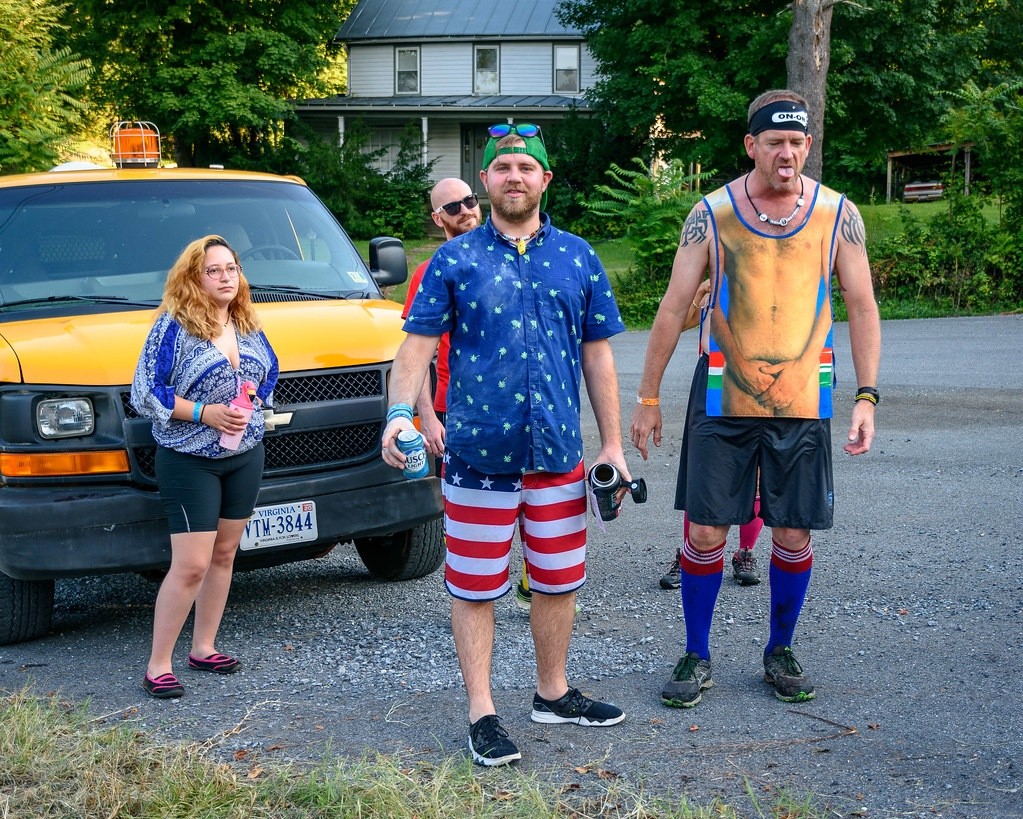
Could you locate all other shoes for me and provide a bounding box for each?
[188,653,242,674]
[515,580,580,615]
[144,672,185,697]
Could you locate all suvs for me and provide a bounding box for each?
[0,119,446,649]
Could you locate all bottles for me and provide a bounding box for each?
[588,462,647,521]
[219,381,257,451]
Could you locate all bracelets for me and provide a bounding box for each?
[192,401,206,424]
[636,395,660,406]
[386,403,414,423]
[854,387,880,405]
[691,296,707,309]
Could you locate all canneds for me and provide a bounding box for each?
[396,430,428,479]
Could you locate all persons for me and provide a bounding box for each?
[130,234,280,699]
[401,177,584,616]
[655,277,765,590]
[628,89,882,707]
[381,123,635,766]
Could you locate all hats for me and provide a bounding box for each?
[481,128,550,212]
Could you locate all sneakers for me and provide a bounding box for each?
[763,646,816,703]
[659,548,682,588]
[661,652,714,708]
[531,685,626,726]
[467,715,521,766]
[732,546,761,586]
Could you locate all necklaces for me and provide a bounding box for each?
[499,228,539,255]
[745,171,804,226]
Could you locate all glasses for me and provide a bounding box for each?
[434,193,478,216]
[203,263,242,280]
[484,123,545,148]
